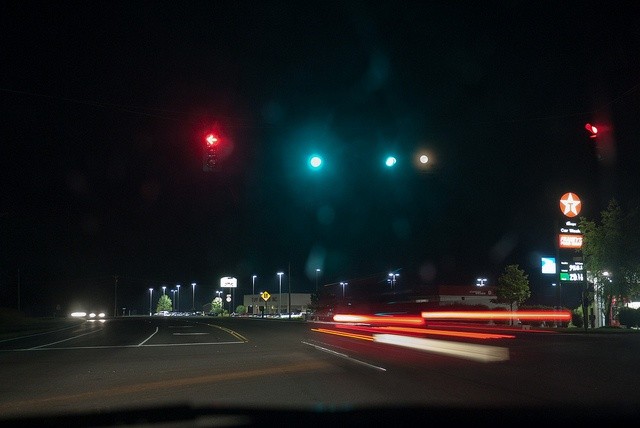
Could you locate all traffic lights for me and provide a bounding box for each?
[203,134,218,171]
[585,123,600,139]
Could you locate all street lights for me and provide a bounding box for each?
[277,272,284,304]
[253,276,257,302]
[149,288,153,317]
[192,283,196,308]
[340,282,348,298]
[316,269,321,291]
[171,290,177,309]
[162,287,166,295]
[176,285,180,311]
